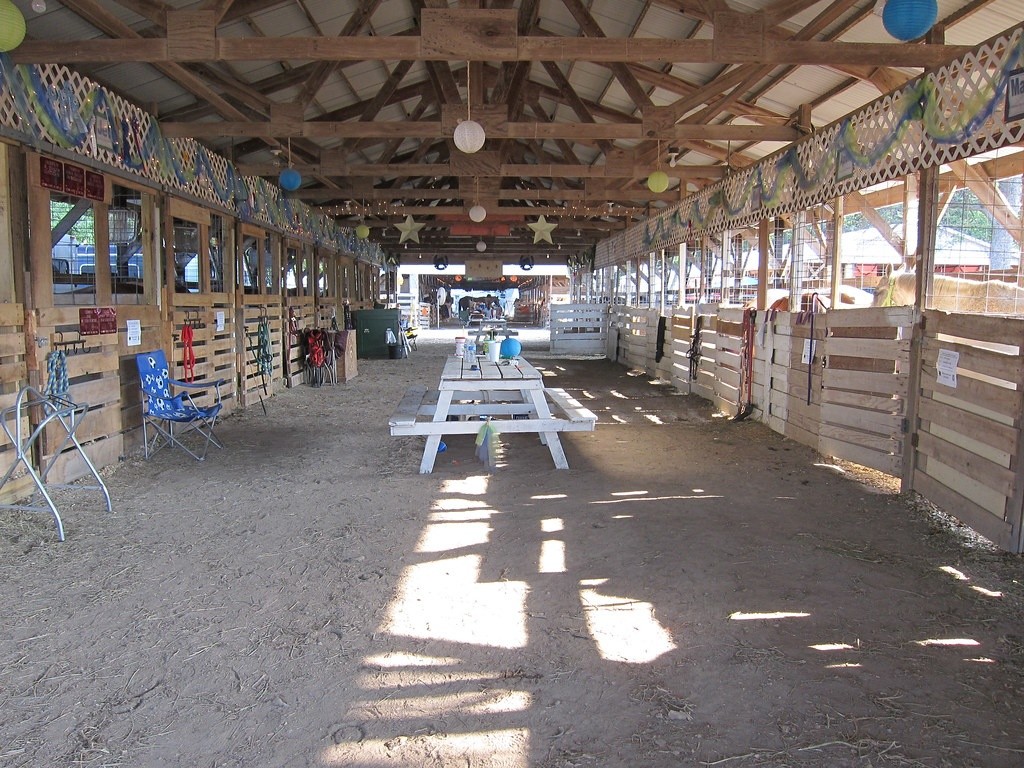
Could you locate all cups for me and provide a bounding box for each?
[455,337,466,356]
[488,341,501,362]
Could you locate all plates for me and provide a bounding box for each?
[454,353,503,359]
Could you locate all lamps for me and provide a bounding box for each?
[344,199,352,211]
[419,254,422,259]
[607,200,614,212]
[269,145,283,167]
[31,0,46,13]
[668,147,679,167]
[404,243,407,249]
[381,228,387,236]
[558,242,561,249]
[576,229,582,237]
[546,254,550,258]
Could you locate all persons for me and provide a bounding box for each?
[455,298,458,310]
[482,304,491,318]
[496,291,500,298]
[501,298,507,317]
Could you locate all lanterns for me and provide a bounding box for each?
[510,276,517,281]
[453,121,485,154]
[455,276,462,281]
[279,169,301,191]
[648,171,668,193]
[501,339,522,358]
[356,225,369,239]
[476,241,486,252]
[0,0,26,52]
[469,206,486,222]
[882,0,938,41]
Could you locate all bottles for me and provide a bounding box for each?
[463,338,476,363]
[482,334,490,353]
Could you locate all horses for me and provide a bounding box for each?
[869,261,1024,315]
[747,285,874,310]
[459,296,500,319]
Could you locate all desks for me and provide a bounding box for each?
[468,330,518,345]
[465,313,486,327]
[419,354,569,474]
[479,319,508,330]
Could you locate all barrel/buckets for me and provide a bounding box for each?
[388,345,402,359]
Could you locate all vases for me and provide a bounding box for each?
[387,345,404,359]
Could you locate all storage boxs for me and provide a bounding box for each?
[309,329,360,383]
[349,309,402,359]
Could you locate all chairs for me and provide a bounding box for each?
[136,350,225,461]
[408,334,417,351]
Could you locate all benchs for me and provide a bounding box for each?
[387,386,428,427]
[544,387,598,424]
